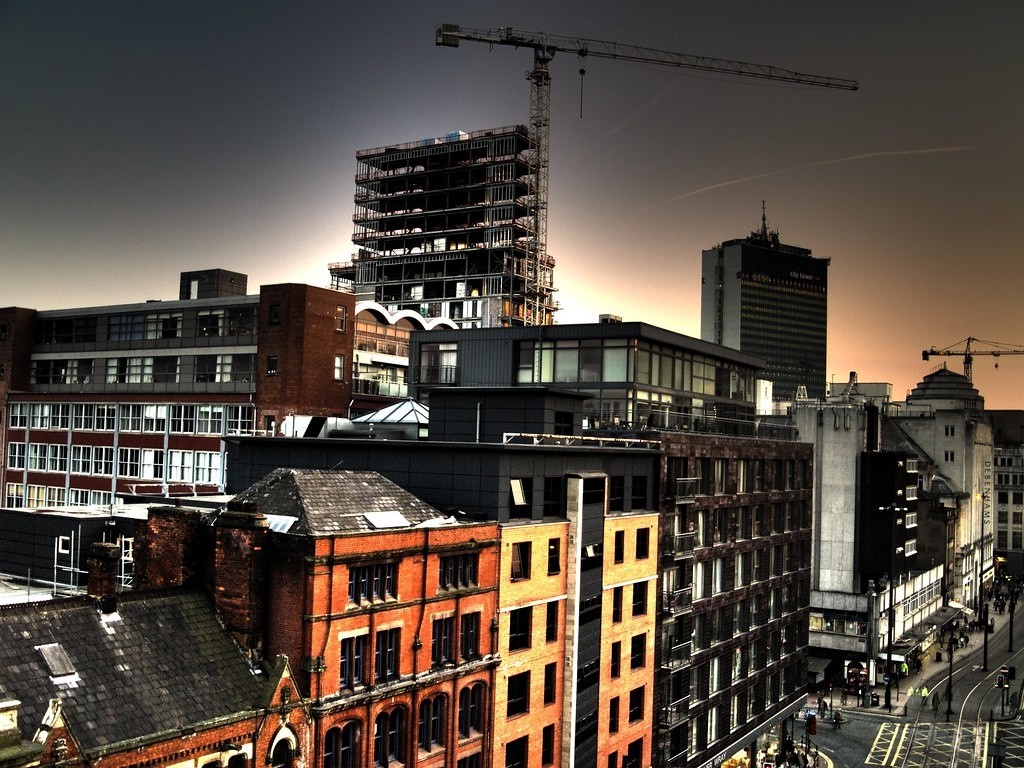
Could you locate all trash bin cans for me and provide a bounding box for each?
[935,651,943,662]
[871,693,880,706]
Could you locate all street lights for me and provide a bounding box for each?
[945,616,965,715]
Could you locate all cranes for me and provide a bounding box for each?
[432,23,863,325]
[922,334,1024,393]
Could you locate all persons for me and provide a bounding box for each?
[915,572,1024,674]
[762,686,848,768]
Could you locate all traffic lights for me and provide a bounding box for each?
[997,675,1004,689]
